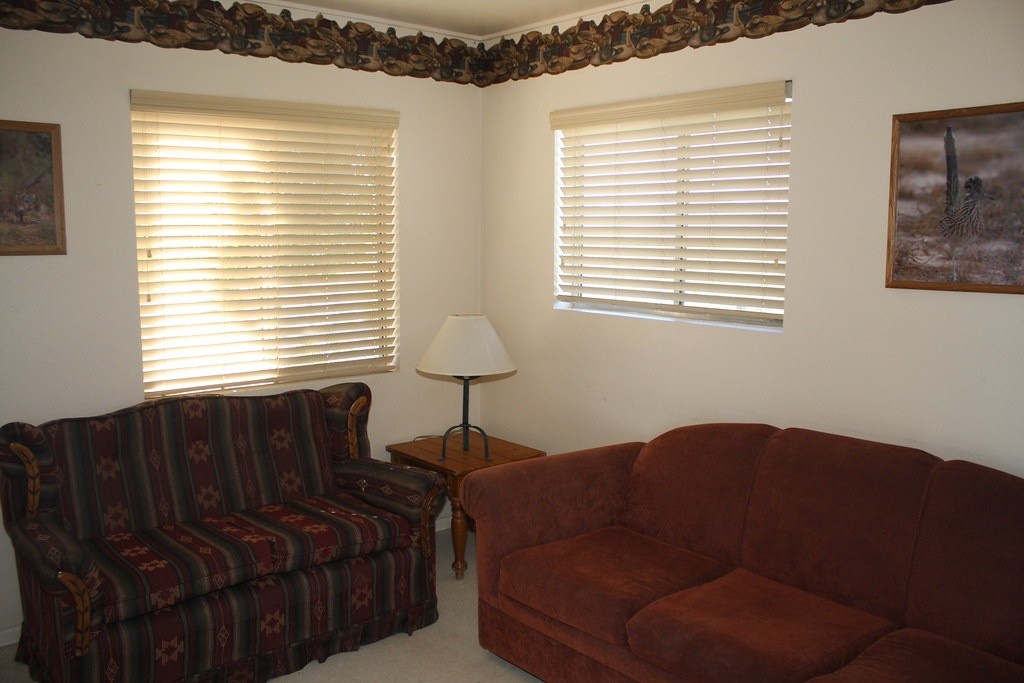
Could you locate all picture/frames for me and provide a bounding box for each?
[884,101,1024,296]
[0,119,68,257]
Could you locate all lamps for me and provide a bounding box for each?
[416,313,518,462]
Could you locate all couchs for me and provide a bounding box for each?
[456,423,1024,683]
[0,380,449,683]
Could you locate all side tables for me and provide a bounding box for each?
[387,430,548,580]
